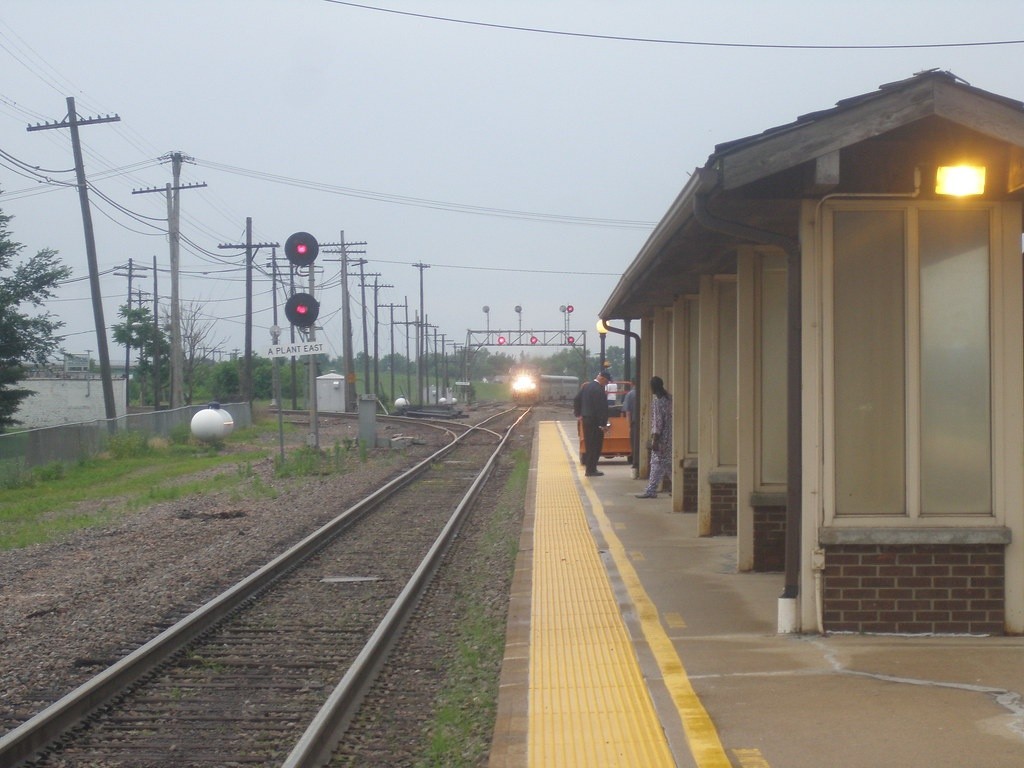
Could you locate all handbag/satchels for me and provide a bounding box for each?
[574,387,584,417]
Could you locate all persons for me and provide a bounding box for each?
[625,377,637,468]
[636,376,672,498]
[575,371,611,476]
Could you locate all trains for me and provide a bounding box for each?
[509,364,580,406]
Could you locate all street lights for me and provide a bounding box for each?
[596,319,610,392]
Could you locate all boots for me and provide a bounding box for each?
[631,467,639,480]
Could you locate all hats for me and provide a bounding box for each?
[599,371,611,381]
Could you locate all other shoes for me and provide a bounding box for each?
[585,470,604,477]
[634,492,657,498]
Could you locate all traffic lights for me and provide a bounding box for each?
[285,292,320,327]
[284,232,319,266]
